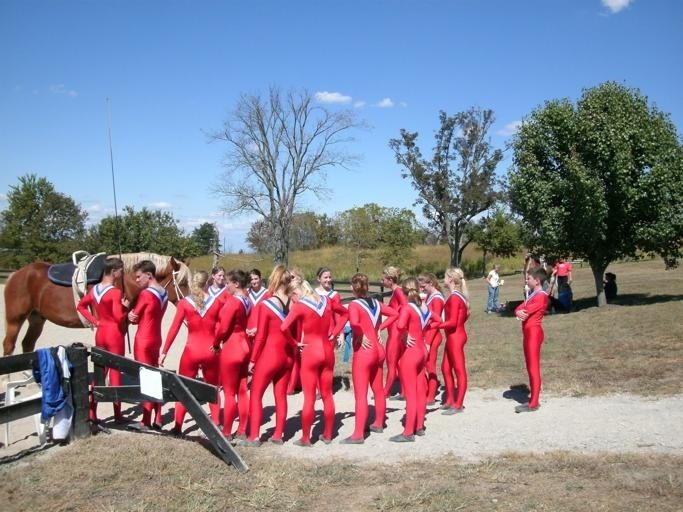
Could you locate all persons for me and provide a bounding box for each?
[127,261,169,431]
[158,265,349,443]
[79,260,132,426]
[515,268,548,413]
[419,272,445,411]
[595,273,617,302]
[389,278,442,442]
[524,252,573,314]
[340,274,399,443]
[381,266,408,398]
[486,264,502,314]
[439,267,469,415]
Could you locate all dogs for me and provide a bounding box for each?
[2,251,192,391]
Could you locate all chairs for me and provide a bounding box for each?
[366,423,382,432]
[115,415,163,434]
[389,396,404,401]
[317,432,331,444]
[264,436,283,445]
[515,401,541,413]
[440,402,463,415]
[293,439,311,445]
[167,428,182,438]
[219,424,261,447]
[390,428,424,442]
[340,437,365,443]
[91,418,101,434]
